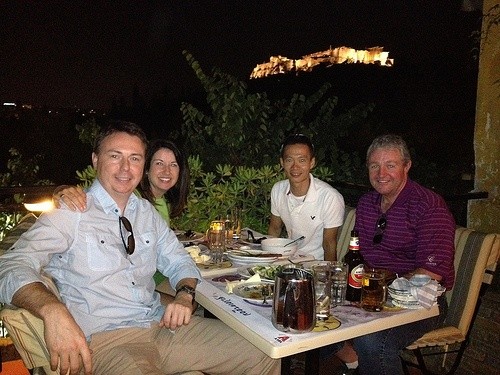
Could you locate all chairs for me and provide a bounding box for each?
[0,276,76,375]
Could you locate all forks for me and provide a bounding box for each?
[247,268,272,280]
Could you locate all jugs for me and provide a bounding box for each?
[271,268,316,334]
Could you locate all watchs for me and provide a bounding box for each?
[175,284,196,304]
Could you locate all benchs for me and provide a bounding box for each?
[336,205,500,375]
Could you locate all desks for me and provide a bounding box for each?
[173,228,440,358]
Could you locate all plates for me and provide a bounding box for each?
[227,236,278,265]
[174,231,205,243]
[301,260,331,271]
[232,282,274,306]
[236,263,278,282]
[182,243,208,254]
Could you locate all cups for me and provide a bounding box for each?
[206,221,225,252]
[360,268,387,311]
[222,207,241,243]
[327,261,348,306]
[313,266,331,320]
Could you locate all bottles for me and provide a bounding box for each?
[342,230,365,302]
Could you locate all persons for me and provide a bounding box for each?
[268,136,358,375]
[0,122,281,375]
[334,135,455,375]
[51,141,204,318]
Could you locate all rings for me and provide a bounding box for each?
[60,194,64,198]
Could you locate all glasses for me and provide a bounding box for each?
[373,217,387,245]
[119,216,135,255]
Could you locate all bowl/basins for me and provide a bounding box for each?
[260,237,298,259]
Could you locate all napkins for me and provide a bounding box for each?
[184,245,210,262]
[227,273,261,293]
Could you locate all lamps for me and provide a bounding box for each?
[23,194,52,212]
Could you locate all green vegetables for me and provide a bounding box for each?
[255,265,281,279]
